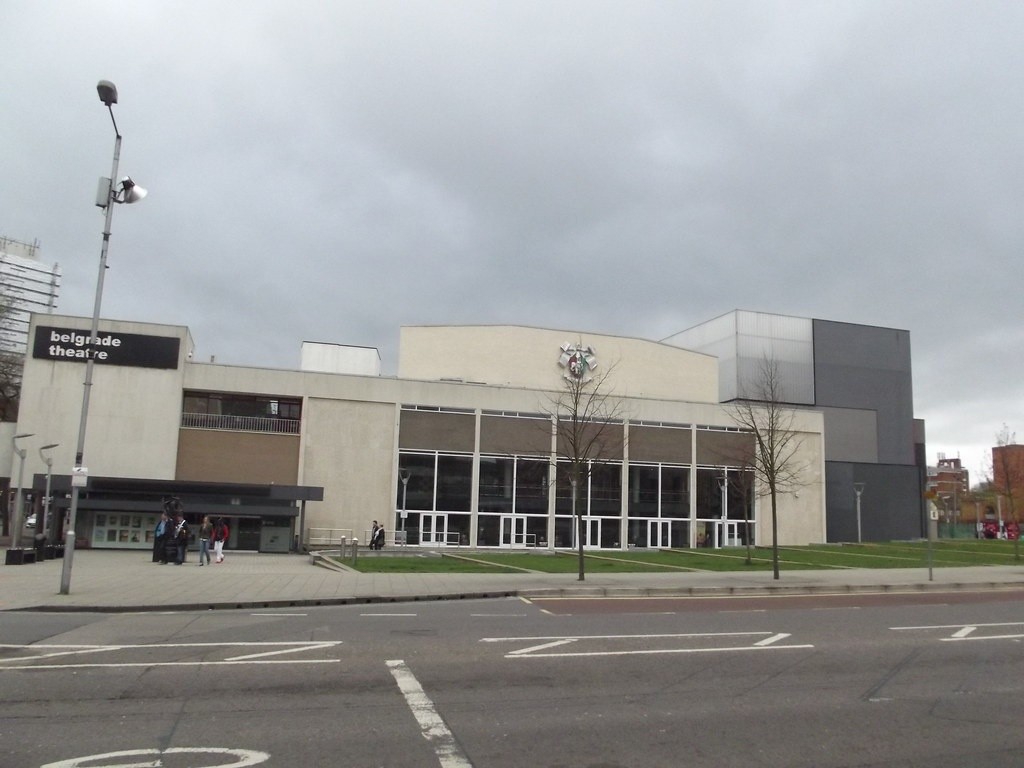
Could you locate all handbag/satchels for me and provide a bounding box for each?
[378,539,384,546]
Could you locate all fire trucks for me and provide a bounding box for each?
[976,520,1021,541]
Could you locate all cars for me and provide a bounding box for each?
[26,514,37,529]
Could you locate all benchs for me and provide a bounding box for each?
[45,545,65,560]
[5,548,37,565]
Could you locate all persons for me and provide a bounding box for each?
[153,512,189,565]
[198,516,213,565]
[212,516,228,563]
[369,520,385,550]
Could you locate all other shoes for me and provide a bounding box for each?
[160,561,168,565]
[215,561,221,563]
[199,564,204,566]
[221,556,224,561]
[175,561,182,565]
[207,560,210,565]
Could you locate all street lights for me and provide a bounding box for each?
[12,432,34,548]
[38,443,61,535]
[853,483,865,544]
[717,476,730,548]
[398,467,412,549]
[58,80,123,595]
